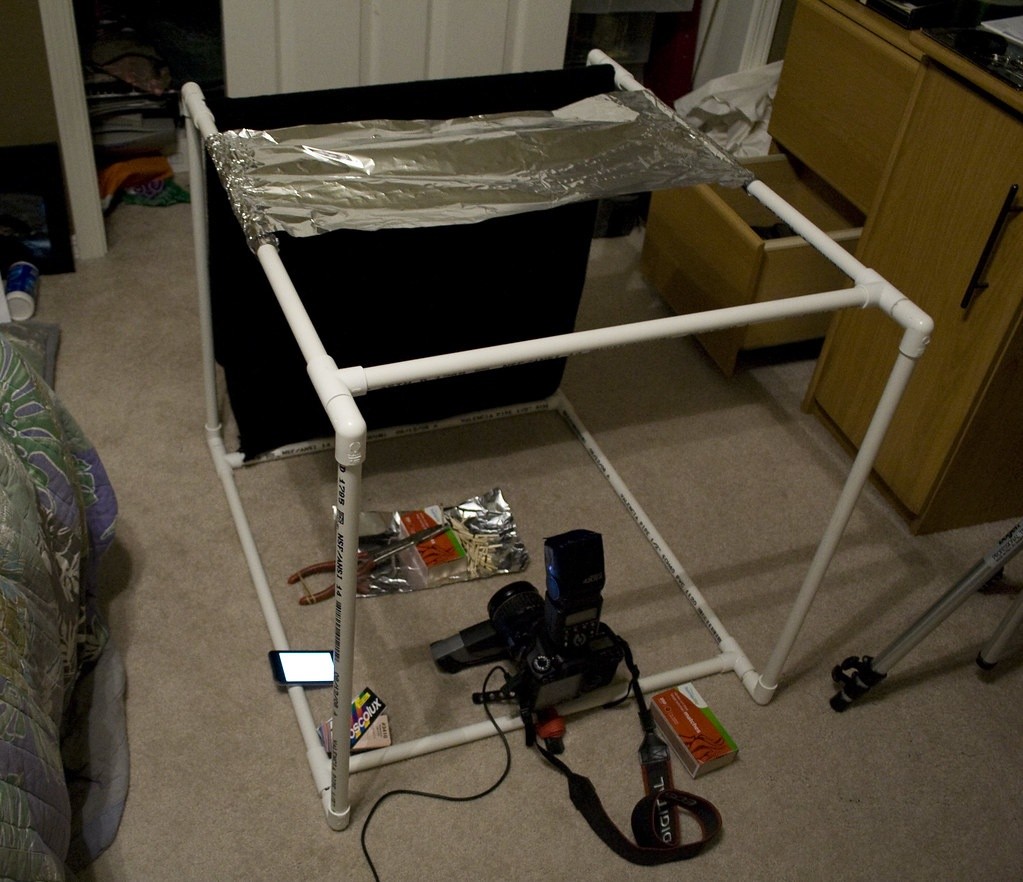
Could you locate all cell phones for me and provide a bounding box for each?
[268,650,335,687]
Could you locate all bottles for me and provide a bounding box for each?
[4,262,40,321]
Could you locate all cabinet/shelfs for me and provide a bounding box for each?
[639,0,1023,534]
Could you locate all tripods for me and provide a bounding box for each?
[829,525,1023,714]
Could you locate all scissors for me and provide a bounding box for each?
[287,521,450,606]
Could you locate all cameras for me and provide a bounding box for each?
[486,527,624,712]
